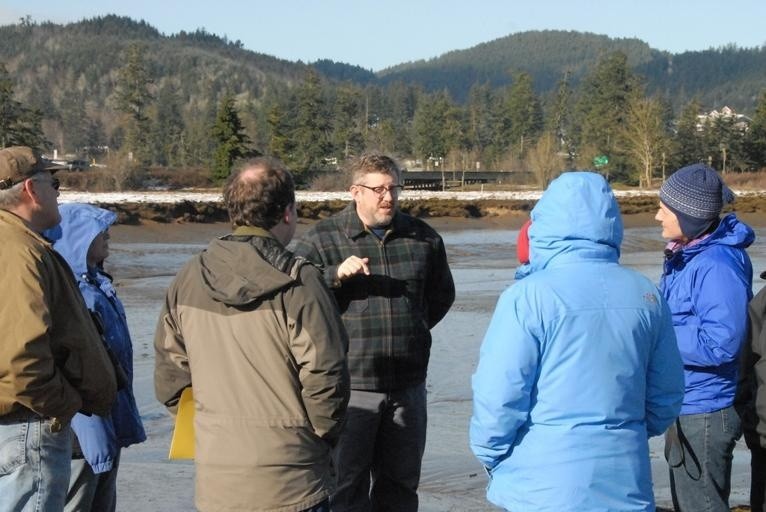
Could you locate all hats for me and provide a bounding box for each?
[660,164,734,240]
[0,146,70,191]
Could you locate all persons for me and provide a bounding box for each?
[155,162,350,512]
[291,154,455,511]
[48,203,147,512]
[0,146,127,512]
[469,170,685,511]
[733,270,766,510]
[653,161,757,512]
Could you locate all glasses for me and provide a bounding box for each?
[34,176,60,190]
[356,184,405,196]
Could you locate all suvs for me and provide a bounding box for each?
[62,158,90,174]
